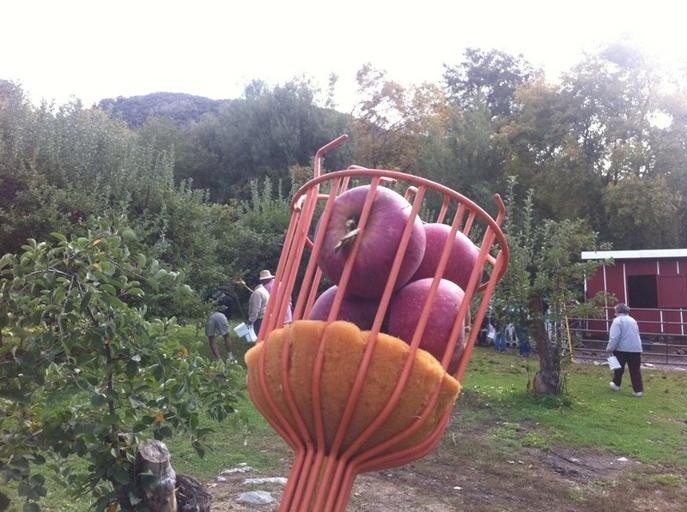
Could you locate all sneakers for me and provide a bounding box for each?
[632,392,642,396]
[609,381,620,391]
[494,347,531,358]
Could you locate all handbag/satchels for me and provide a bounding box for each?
[232,321,258,343]
[606,353,621,371]
[487,332,495,338]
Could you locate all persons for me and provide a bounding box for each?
[247,270,292,338]
[490,305,537,356]
[606,303,642,397]
[205,305,237,364]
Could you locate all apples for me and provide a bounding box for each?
[309,184,478,375]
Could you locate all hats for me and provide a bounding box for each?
[258,270,275,280]
